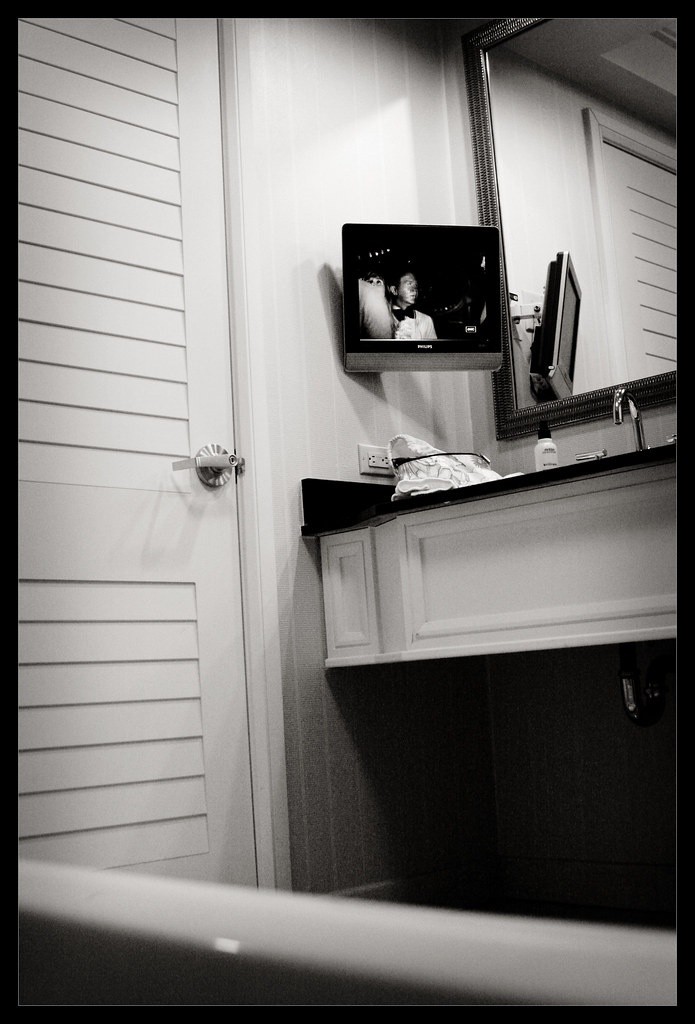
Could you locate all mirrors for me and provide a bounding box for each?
[463,17,677,443]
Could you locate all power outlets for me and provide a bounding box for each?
[358,443,399,477]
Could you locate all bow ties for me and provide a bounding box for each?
[391,304,414,322]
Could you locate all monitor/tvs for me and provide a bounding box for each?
[342,223,504,374]
[529,252,583,402]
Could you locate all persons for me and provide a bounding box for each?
[384,269,438,340]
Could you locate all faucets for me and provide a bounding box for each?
[612,387,646,453]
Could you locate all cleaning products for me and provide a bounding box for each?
[534,421,558,474]
